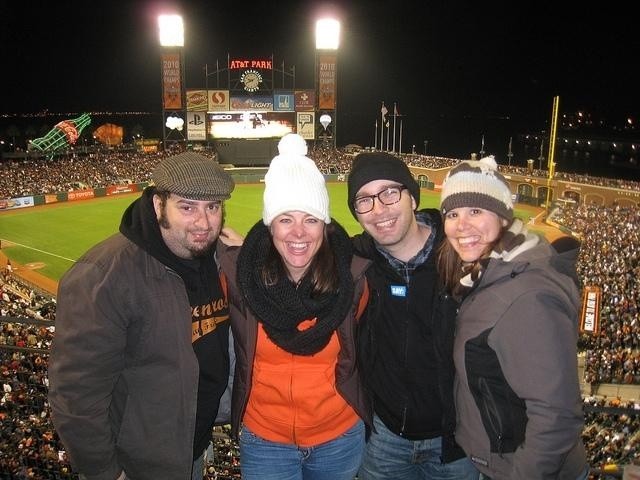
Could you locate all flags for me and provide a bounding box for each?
[380,104,388,117]
[395,107,400,117]
[385,122,389,127]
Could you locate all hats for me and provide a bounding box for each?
[261,132,331,227]
[347,151,422,222]
[150,150,235,202]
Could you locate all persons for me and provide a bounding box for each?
[550,197,640,479]
[217,153,480,479]
[253,113,261,128]
[0,136,216,194]
[48,153,236,480]
[438,156,589,478]
[306,143,353,174]
[394,152,460,168]
[242,109,250,128]
[497,165,639,191]
[214,133,377,478]
[0,260,79,480]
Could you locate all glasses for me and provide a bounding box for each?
[439,157,515,222]
[352,185,409,214]
